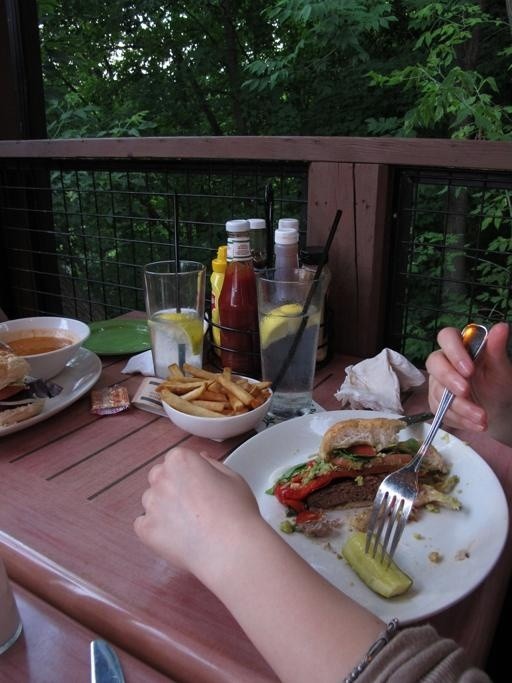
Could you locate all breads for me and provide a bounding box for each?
[321,417,406,458]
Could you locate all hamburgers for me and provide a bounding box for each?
[0,355,43,425]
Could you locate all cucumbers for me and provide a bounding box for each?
[342,533,411,598]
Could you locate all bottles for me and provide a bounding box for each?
[298,244,336,369]
[209,216,302,382]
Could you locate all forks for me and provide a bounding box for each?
[364,322,492,568]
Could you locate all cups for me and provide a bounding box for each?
[259,263,324,410]
[1,561,25,655]
[149,307,210,338]
[141,261,209,387]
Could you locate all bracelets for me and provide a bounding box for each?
[344,617,399,682]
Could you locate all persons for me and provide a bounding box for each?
[131,319,511,682]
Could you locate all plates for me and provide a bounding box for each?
[1,345,103,442]
[219,406,511,628]
[83,318,154,356]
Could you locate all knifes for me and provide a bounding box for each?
[87,638,124,683]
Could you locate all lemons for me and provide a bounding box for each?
[147,314,203,355]
[260,303,320,347]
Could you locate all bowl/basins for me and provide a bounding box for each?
[160,373,272,443]
[0,314,91,383]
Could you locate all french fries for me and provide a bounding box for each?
[154,361,272,417]
